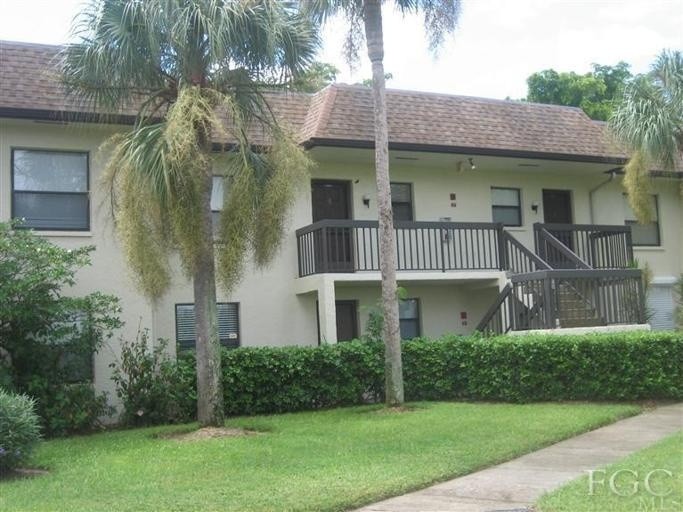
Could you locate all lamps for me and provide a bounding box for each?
[467,158,478,171]
[531,199,538,215]
[363,193,371,208]
